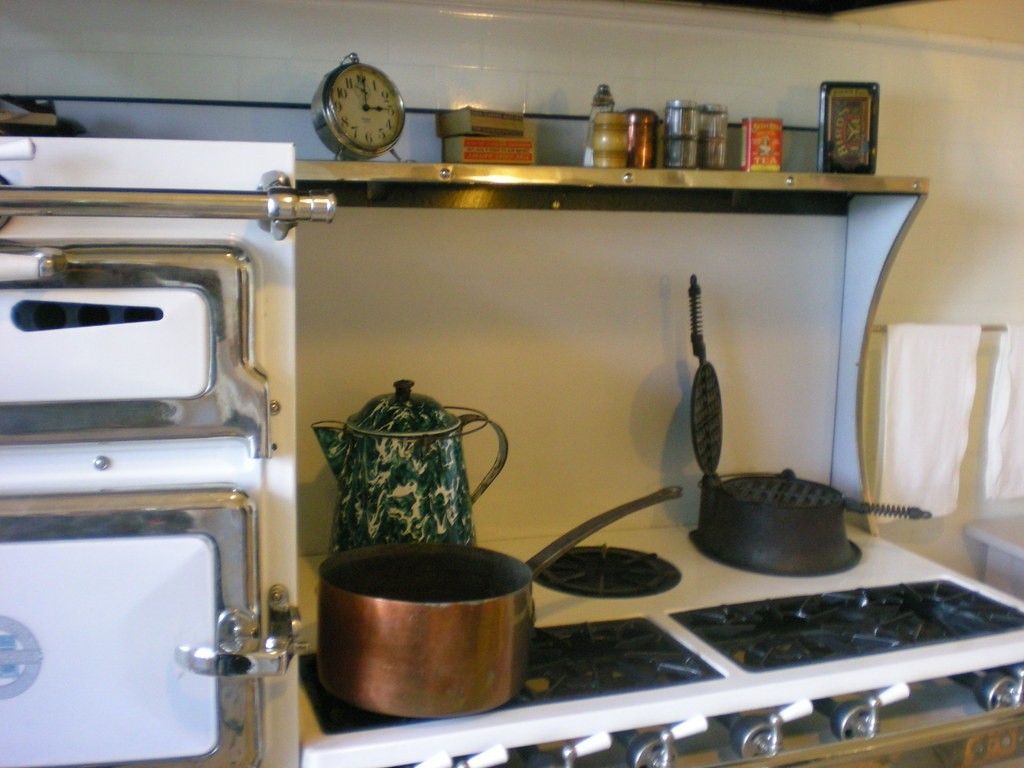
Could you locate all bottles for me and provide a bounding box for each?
[623,107,658,168]
[593,113,631,168]
[697,103,728,169]
[582,84,614,166]
[663,100,699,169]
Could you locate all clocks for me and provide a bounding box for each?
[310,53,406,161]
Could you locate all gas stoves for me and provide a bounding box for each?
[299,526,1024,768]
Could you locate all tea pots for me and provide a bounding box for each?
[310,379,508,546]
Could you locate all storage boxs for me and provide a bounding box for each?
[436,105,524,138]
[442,135,536,164]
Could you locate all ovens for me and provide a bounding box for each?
[1,135,337,768]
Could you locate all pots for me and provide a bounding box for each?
[315,483,681,719]
[686,273,932,575]
